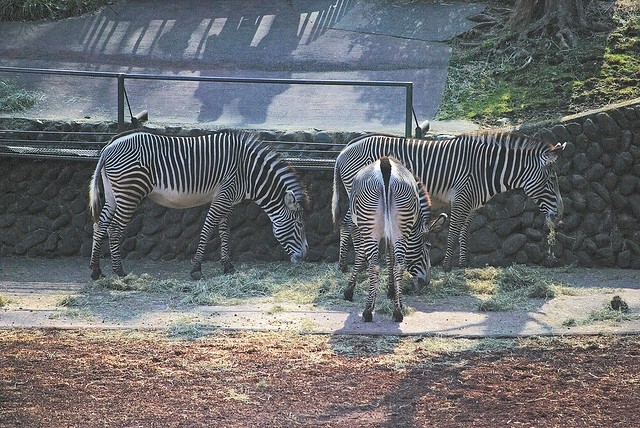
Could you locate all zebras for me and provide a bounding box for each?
[332,131,567,274]
[343,155,449,322]
[88,127,311,279]
